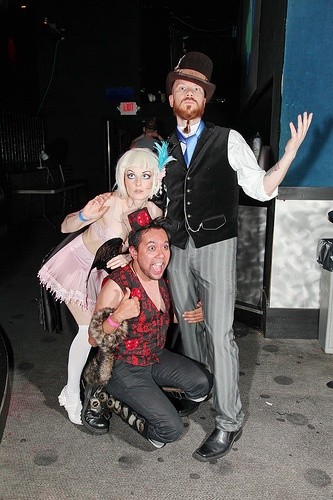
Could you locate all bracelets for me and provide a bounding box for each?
[78,210,88,222]
[107,316,121,328]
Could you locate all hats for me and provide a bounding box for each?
[165,52,216,104]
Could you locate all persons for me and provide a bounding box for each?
[37,131,211,449]
[149,52,313,461]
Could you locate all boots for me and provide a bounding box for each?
[162,385,207,402]
[80,385,166,449]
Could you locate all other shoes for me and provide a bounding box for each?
[57,385,84,426]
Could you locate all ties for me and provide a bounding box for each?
[174,120,205,169]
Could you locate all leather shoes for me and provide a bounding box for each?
[178,400,202,417]
[192,428,242,461]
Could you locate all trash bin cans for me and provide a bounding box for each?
[316,238,333,354]
